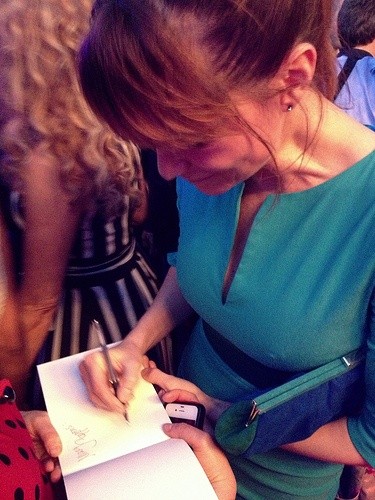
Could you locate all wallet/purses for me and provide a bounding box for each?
[218,346,366,458]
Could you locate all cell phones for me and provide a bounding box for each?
[163,400,204,452]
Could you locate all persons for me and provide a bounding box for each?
[0,0,236,500]
[329,0,375,131]
[74,0,375,500]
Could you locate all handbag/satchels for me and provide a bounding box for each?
[0,379,54,500]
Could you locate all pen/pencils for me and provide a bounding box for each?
[90,319,131,422]
